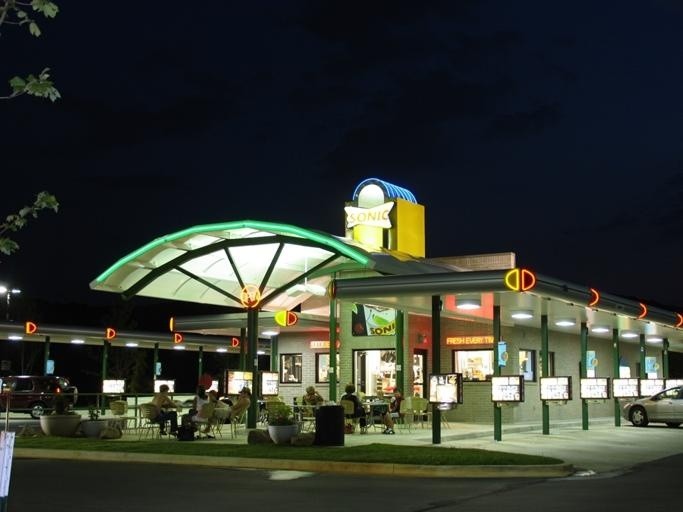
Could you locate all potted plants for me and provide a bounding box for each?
[260,403,300,445]
[39,391,82,437]
[78,405,108,437]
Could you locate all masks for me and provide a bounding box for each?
[394,393,400,398]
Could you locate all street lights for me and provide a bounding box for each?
[0,286,21,322]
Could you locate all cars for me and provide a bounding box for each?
[622,384,683,430]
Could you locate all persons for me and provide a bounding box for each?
[341,384,367,434]
[149,385,179,436]
[303,386,323,417]
[183,385,251,433]
[383,388,406,434]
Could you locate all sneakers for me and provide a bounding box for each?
[385,427,392,433]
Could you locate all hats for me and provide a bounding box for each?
[206,390,217,397]
[239,387,250,393]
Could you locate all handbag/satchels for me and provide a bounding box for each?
[176,426,197,441]
[344,423,355,434]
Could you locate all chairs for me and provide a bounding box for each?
[109,400,135,435]
[115,400,137,433]
[291,389,449,436]
[136,396,249,448]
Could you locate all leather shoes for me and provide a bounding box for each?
[171,431,177,436]
[160,431,167,435]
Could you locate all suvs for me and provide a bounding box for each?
[1,375,78,420]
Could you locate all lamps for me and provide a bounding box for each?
[454,297,481,311]
[509,309,535,321]
[620,329,638,338]
[645,334,664,343]
[591,324,610,334]
[552,316,576,327]
[285,247,327,298]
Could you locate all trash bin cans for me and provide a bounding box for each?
[314,406,344,445]
